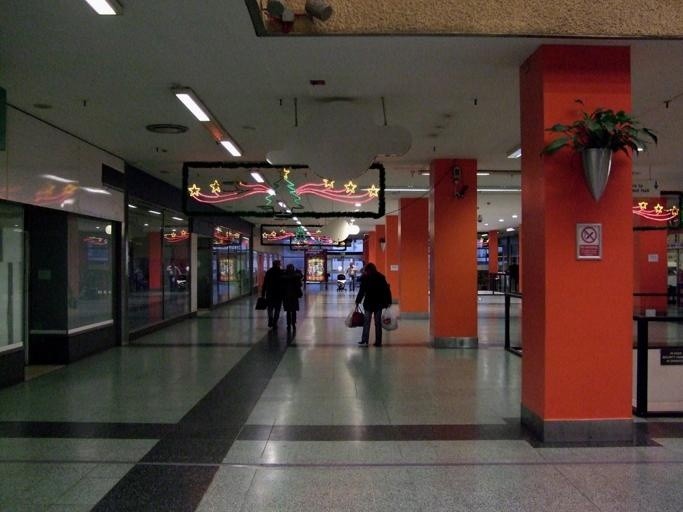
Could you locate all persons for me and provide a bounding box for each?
[281,264,303,329]
[262,260,284,329]
[355,263,392,347]
[507,258,519,293]
[346,263,357,292]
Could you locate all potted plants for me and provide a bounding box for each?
[540,107,659,201]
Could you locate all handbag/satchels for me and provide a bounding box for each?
[297,288,303,298]
[352,312,364,327]
[256,297,266,309]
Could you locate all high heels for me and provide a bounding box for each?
[358,339,381,347]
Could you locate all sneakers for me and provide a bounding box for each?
[268,318,277,327]
[288,321,295,329]
